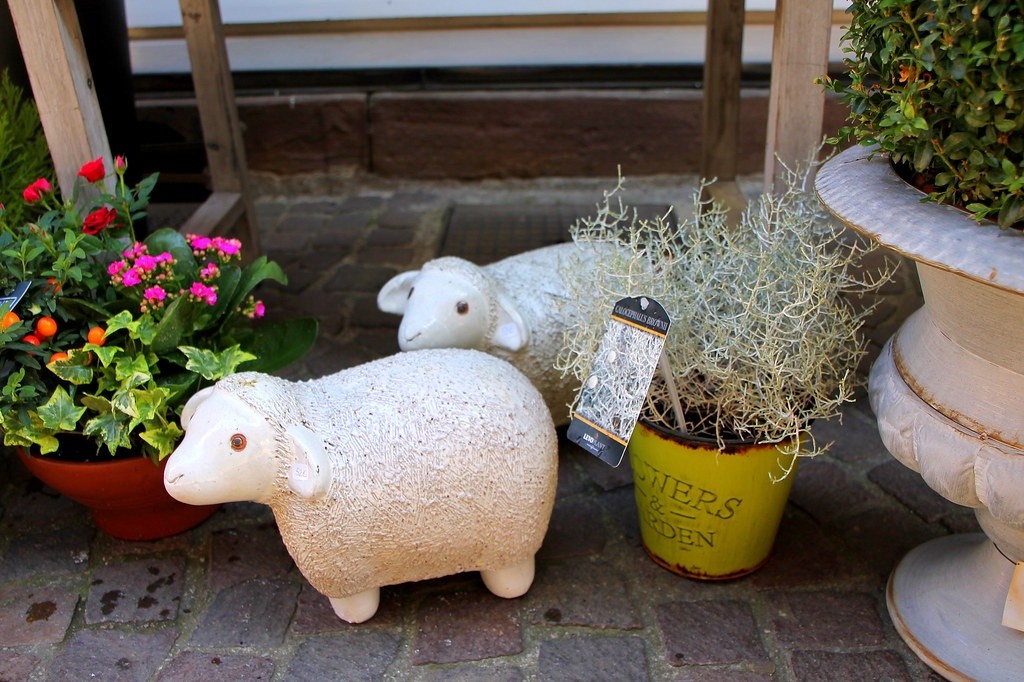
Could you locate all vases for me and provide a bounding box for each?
[14,438,225,545]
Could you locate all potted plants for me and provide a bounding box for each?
[537,131,901,585]
[815,0,1024,682]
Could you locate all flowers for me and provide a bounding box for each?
[0,153,318,470]
